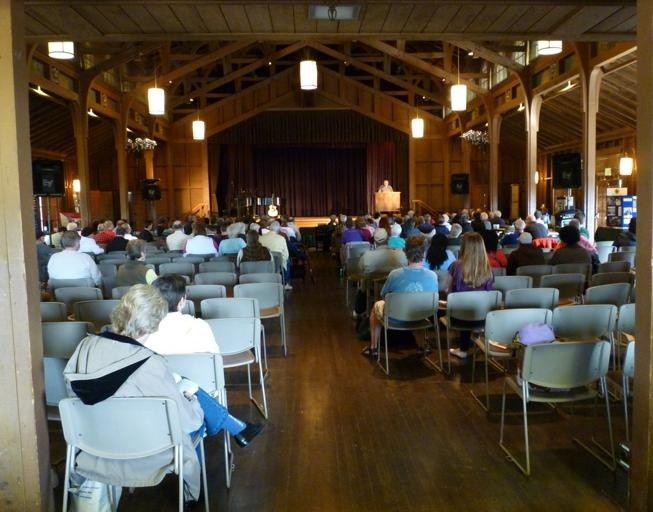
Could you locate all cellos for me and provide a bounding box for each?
[268,193,279,218]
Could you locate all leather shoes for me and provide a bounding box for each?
[234,420,264,447]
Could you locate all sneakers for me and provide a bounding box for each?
[284,283,292,291]
[449,347,467,359]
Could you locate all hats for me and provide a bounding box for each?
[372,228,387,243]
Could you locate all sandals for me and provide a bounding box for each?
[360,345,377,359]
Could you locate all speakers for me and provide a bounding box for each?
[33,160,64,196]
[143,179,160,200]
[452,173,469,194]
[553,153,581,188]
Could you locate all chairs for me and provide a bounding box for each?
[498,342,617,474]
[203,318,269,421]
[39,319,94,423]
[56,395,209,512]
[316,212,634,411]
[592,339,635,470]
[156,352,234,490]
[37,213,289,417]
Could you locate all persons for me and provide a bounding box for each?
[594,216,636,248]
[327,207,551,355]
[60,282,265,512]
[137,273,220,354]
[155,213,302,290]
[549,212,599,276]
[36,219,158,291]
[376,179,394,192]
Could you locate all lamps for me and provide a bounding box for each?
[190,101,205,140]
[449,47,468,112]
[71,179,80,192]
[299,47,318,92]
[146,65,166,116]
[44,40,76,61]
[536,41,562,56]
[410,98,424,139]
[619,156,633,176]
